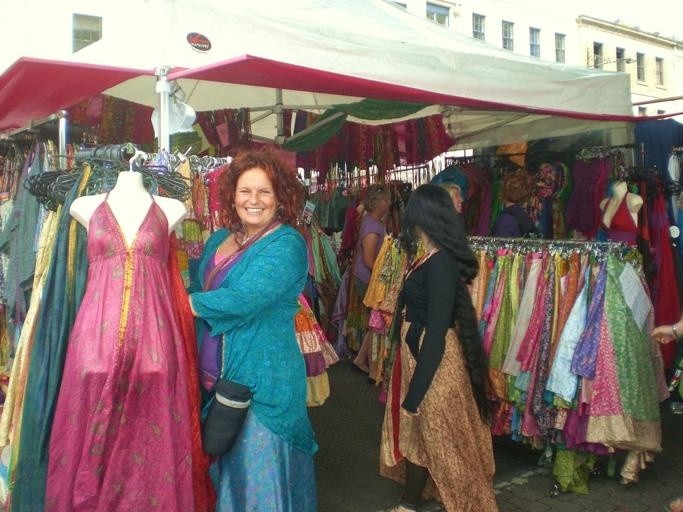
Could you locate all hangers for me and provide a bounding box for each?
[21,141,227,213]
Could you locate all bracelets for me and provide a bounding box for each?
[672,325,681,340]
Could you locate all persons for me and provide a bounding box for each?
[351,184,391,379]
[600,182,643,244]
[387,184,498,512]
[183,154,319,512]
[45,171,215,512]
[651,318,683,512]
